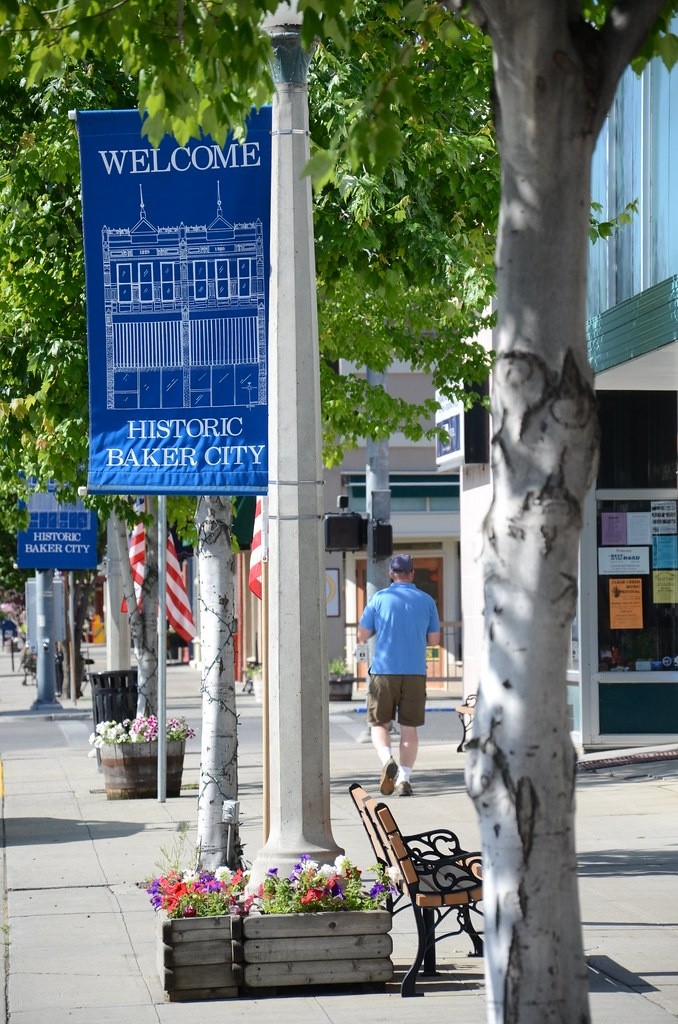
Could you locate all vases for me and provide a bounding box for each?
[100,740,186,800]
[243,904,393,988]
[158,911,244,1003]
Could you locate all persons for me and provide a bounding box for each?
[359,553,441,796]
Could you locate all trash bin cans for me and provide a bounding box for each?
[55,651,64,695]
[86,670,139,773]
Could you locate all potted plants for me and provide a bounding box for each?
[326,658,353,702]
[244,665,264,702]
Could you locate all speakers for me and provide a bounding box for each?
[324,514,364,551]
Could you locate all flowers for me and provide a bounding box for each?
[242,853,398,914]
[135,833,248,919]
[87,715,196,748]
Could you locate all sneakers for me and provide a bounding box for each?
[380,755,398,796]
[395,781,411,796]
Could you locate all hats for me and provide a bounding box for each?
[390,553,412,570]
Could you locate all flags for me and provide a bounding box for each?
[248,495,263,602]
[120,496,196,641]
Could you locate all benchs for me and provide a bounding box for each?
[349,782,487,998]
